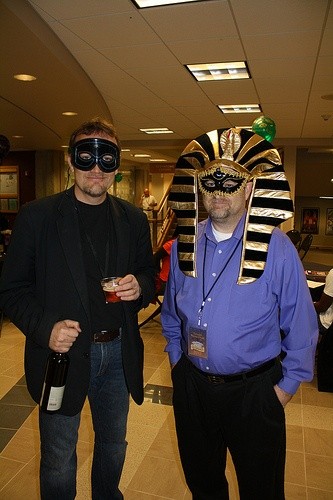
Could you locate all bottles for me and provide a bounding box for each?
[40,352,68,413]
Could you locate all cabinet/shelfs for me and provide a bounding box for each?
[0,165,35,225]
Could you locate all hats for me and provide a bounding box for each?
[167,128,295,285]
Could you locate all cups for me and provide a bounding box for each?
[101,277,121,303]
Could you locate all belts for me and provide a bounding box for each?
[185,353,281,385]
[91,327,121,343]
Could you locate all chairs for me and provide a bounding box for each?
[286,230,313,261]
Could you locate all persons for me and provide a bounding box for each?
[154,237,177,296]
[314,266,333,330]
[159,127,319,500]
[0,116,158,500]
[138,184,157,241]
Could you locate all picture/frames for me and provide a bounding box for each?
[325,208,333,236]
[301,207,320,234]
